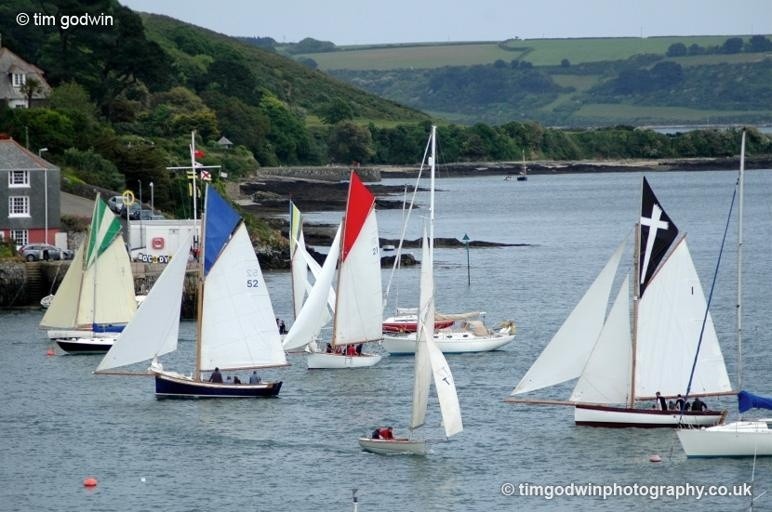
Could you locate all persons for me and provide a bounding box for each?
[372,426,393,440]
[326,342,362,355]
[250,371,260,384]
[651,392,707,411]
[208,367,241,384]
[275,318,285,334]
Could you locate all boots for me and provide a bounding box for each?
[18,241,73,261]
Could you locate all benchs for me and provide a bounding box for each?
[187,150,227,198]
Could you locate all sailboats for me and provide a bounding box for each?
[42,121,771,459]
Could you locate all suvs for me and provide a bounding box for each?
[18,241,73,261]
